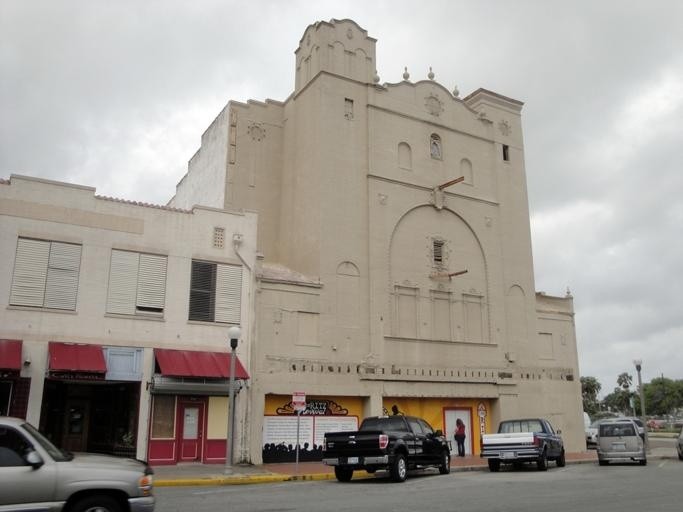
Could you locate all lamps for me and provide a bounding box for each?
[24,355,31,364]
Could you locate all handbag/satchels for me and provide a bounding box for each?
[454,434,466,442]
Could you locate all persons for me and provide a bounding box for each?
[453,418,465,456]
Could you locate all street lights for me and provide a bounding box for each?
[632,358,649,454]
[223,326,241,475]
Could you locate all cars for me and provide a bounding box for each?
[585,417,646,465]
[0,417,154,511]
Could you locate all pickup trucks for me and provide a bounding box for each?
[479,418,565,470]
[322,414,451,482]
